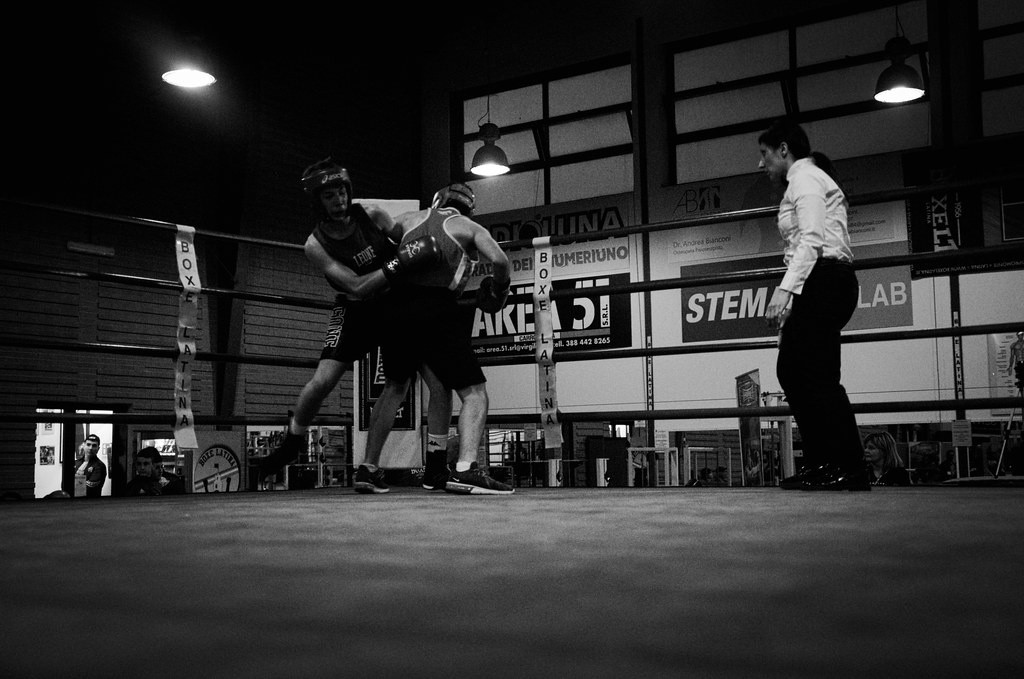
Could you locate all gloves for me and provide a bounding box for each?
[479,275,511,313]
[383,236,442,282]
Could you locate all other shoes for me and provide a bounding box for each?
[779,463,873,492]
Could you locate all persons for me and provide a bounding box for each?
[759,121,875,492]
[45,435,185,498]
[606,441,729,487]
[258,158,456,490]
[46,448,55,466]
[863,429,913,487]
[355,184,515,494]
[746,450,780,487]
[937,450,976,487]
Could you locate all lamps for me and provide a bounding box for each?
[469,67,511,178]
[161,32,218,88]
[873,0,927,104]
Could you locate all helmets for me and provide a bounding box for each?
[299,159,352,197]
[430,183,477,213]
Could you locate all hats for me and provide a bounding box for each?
[85,434,101,445]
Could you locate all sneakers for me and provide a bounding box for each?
[422,469,474,492]
[266,437,308,471]
[447,462,517,495]
[354,464,390,494]
